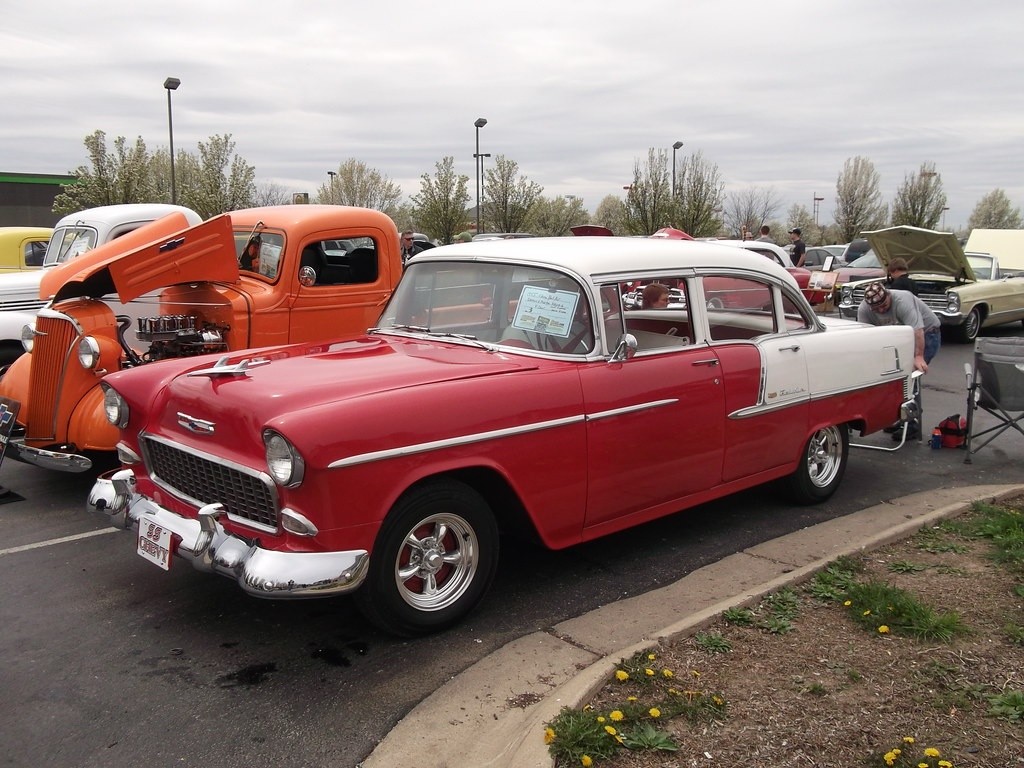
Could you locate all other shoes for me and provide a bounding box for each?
[891,424,919,439]
[884,420,901,433]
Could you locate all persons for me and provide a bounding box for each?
[641,283,670,309]
[754,226,777,259]
[884,258,919,295]
[857,282,942,442]
[399,230,424,270]
[451,232,481,305]
[788,227,806,267]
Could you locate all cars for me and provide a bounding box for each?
[836,224,1024,344]
[86,231,923,643]
[0,203,521,472]
[0,226,82,275]
[0,203,204,374]
[255,217,888,313]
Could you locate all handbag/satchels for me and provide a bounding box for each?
[939,414,967,448]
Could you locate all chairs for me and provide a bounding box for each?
[962,335,1024,463]
[348,247,378,284]
[626,329,688,351]
[302,245,327,285]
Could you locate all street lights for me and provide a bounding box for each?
[474,153,491,234]
[941,206,949,232]
[474,117,487,235]
[327,171,337,205]
[671,141,684,199]
[163,76,182,204]
[813,197,824,226]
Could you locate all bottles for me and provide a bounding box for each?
[933,427,941,436]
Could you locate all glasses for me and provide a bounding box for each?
[404,237,414,241]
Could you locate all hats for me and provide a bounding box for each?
[788,228,801,235]
[865,282,886,306]
[454,232,472,242]
